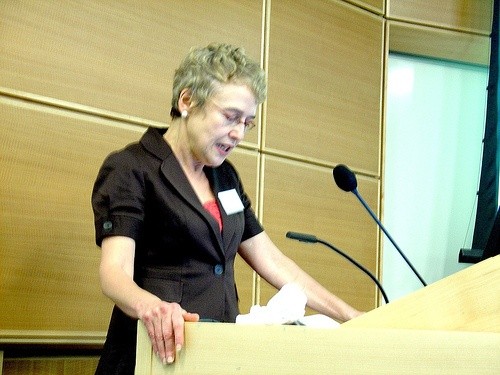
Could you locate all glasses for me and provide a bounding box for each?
[206,99,256,131]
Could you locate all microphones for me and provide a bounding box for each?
[333,164,428,287]
[286,231,390,304]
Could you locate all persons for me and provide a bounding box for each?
[91,43,369,375]
